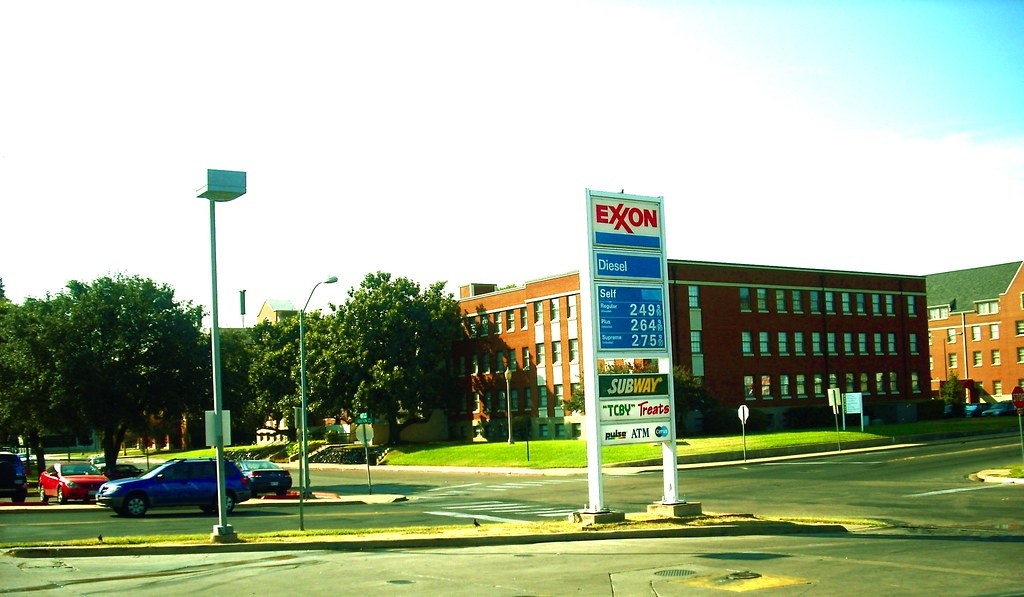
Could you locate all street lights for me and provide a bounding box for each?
[504,366,514,443]
[300,275,338,500]
[944,332,964,382]
[195,167,246,544]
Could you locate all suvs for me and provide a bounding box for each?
[94,457,252,518]
[0,452,28,503]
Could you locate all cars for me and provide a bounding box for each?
[233,460,292,498]
[982,401,1017,417]
[39,461,112,506]
[90,458,145,482]
[17,453,35,465]
[964,403,991,418]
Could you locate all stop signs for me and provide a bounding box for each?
[1012,386,1024,408]
